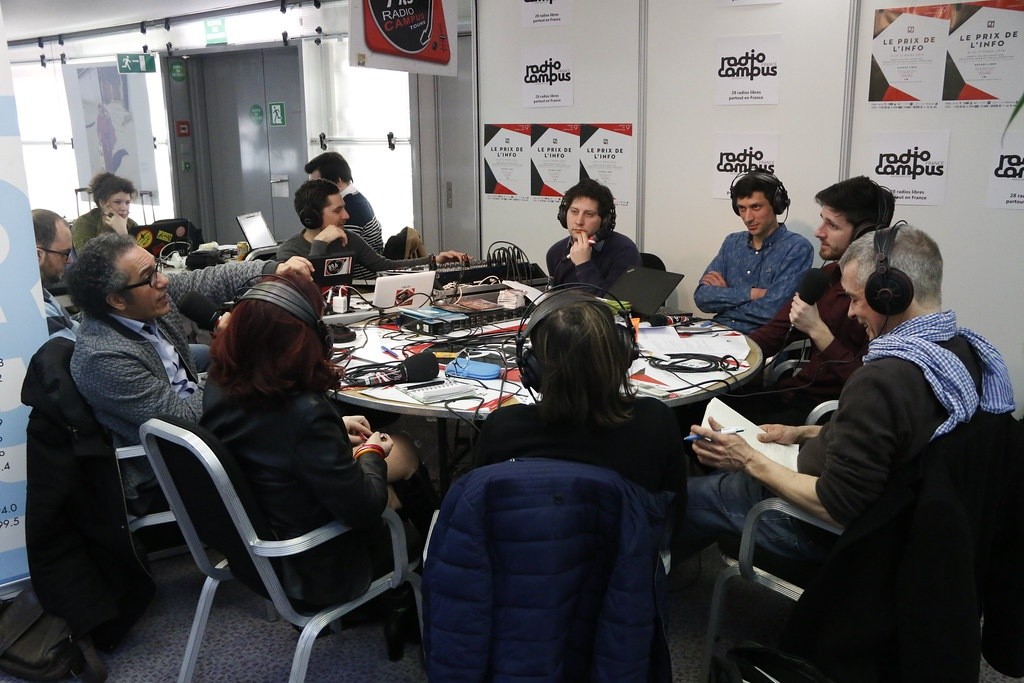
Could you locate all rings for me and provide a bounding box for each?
[108,213,113,217]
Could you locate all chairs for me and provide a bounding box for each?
[138,412,424,683]
[767,359,839,426]
[19,334,276,656]
[420,456,690,683]
[698,411,1024,683]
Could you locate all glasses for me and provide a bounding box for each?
[444,350,507,389]
[121,261,162,291]
[37,247,71,261]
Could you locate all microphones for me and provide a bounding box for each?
[649,314,704,327]
[562,229,613,263]
[177,292,226,340]
[784,268,829,343]
[349,352,440,387]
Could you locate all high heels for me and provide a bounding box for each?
[384,590,421,662]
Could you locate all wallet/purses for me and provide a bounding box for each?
[445,357,500,380]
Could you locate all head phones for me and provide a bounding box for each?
[514,283,640,394]
[209,274,336,363]
[730,169,790,216]
[848,180,894,241]
[299,179,322,229]
[557,186,616,231]
[865,220,914,316]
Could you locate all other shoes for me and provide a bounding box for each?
[717,533,766,560]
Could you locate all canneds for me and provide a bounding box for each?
[236,241,249,261]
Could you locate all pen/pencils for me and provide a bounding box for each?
[380,345,399,359]
[403,380,445,391]
[683,427,745,440]
[701,319,717,327]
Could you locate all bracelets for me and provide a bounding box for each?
[354,443,385,460]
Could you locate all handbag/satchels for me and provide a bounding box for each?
[0,589,107,683]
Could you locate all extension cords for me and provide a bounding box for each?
[320,309,380,325]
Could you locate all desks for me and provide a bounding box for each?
[327,316,765,503]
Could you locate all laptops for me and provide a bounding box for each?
[600,264,694,321]
[303,250,353,314]
[236,211,278,251]
[354,270,436,312]
[129,220,190,262]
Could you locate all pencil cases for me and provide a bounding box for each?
[445,358,501,379]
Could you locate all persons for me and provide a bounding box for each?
[31,209,80,341]
[475,284,688,548]
[276,179,473,295]
[656,167,1015,570]
[71,174,136,259]
[69,232,317,556]
[199,272,438,662]
[545,179,642,297]
[304,152,384,280]
